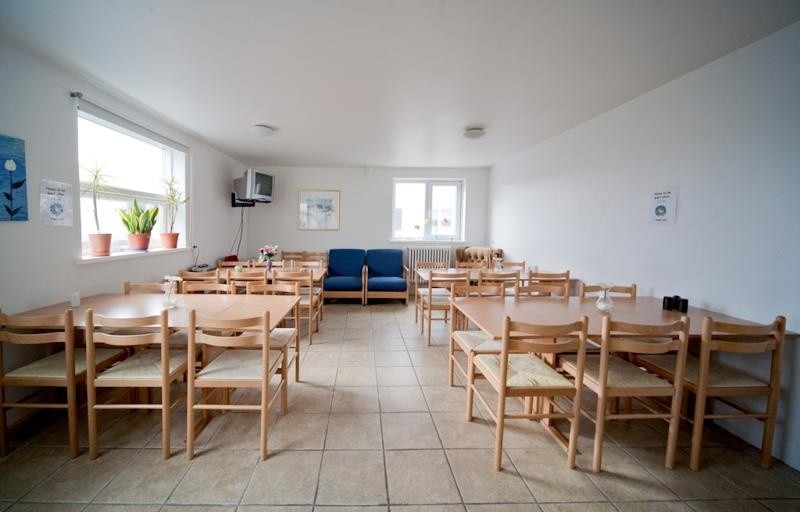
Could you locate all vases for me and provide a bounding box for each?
[265,257,272,271]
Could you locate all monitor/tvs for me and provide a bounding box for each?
[232,168,276,203]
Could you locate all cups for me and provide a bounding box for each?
[70,290,80,307]
[282,260,293,271]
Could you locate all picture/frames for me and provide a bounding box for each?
[298,188,340,230]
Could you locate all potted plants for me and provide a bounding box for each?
[87,169,112,257]
[158,174,190,248]
[118,198,159,250]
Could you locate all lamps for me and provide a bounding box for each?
[250,123,274,139]
[462,128,486,140]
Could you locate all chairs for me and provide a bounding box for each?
[558,316,691,472]
[635,315,787,471]
[528,269,570,297]
[122,280,178,295]
[0,309,126,460]
[473,316,590,473]
[414,262,448,323]
[178,269,221,295]
[86,308,200,460]
[290,259,324,269]
[241,281,300,384]
[580,282,636,418]
[281,250,305,262]
[477,270,520,298]
[252,259,285,269]
[216,259,251,268]
[454,260,491,269]
[186,307,288,461]
[513,282,570,415]
[365,249,410,306]
[323,248,365,305]
[181,280,236,294]
[448,283,506,422]
[226,269,267,296]
[304,252,328,263]
[498,261,526,270]
[418,270,470,346]
[271,269,320,346]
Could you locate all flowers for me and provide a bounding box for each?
[258,245,280,259]
